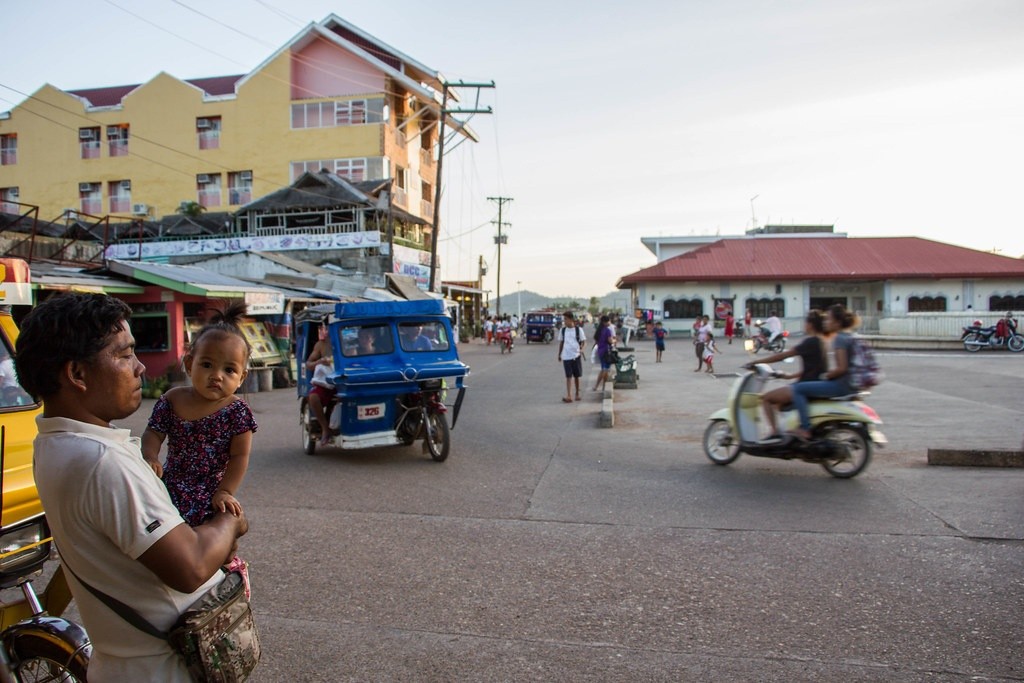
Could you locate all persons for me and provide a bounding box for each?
[306,328,348,445]
[531,314,563,328]
[965,305,973,312]
[349,328,385,355]
[594,311,623,329]
[402,326,432,351]
[574,314,585,327]
[754,310,782,350]
[142,304,258,601]
[724,313,734,344]
[701,334,722,372]
[743,308,752,338]
[692,316,712,372]
[558,312,586,401]
[652,322,668,363]
[742,311,833,443]
[15,291,250,683]
[484,313,527,348]
[784,306,860,438]
[593,316,613,390]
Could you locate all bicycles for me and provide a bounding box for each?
[732,318,744,337]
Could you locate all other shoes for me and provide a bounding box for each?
[761,432,781,444]
[783,429,812,444]
[575,396,581,400]
[562,397,572,402]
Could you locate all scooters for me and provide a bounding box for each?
[748,319,789,354]
[702,357,890,479]
[498,327,516,355]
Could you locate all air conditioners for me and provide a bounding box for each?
[80,183,94,192]
[9,187,18,195]
[198,118,212,129]
[62,207,77,218]
[132,202,148,213]
[79,130,93,139]
[198,173,211,183]
[107,127,118,136]
[241,171,252,180]
[121,180,130,188]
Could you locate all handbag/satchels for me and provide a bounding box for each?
[167,571,261,683]
[609,347,621,365]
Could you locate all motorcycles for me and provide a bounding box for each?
[0,257,93,682]
[526,312,555,344]
[293,298,472,463]
[960,310,1024,353]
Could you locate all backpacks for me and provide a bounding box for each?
[846,339,883,388]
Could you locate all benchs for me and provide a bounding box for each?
[614,354,640,389]
